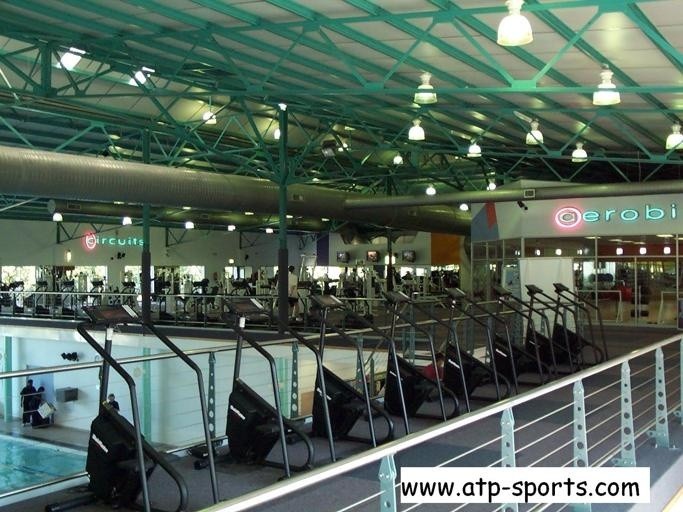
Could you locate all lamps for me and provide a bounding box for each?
[408,0,683,164]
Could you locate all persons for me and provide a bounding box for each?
[20,380,36,426]
[108,394,120,411]
[288,266,302,321]
[29,386,45,426]
[424,353,445,382]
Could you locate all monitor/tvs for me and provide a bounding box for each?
[402,250,415,262]
[336,250,349,263]
[367,250,380,262]
